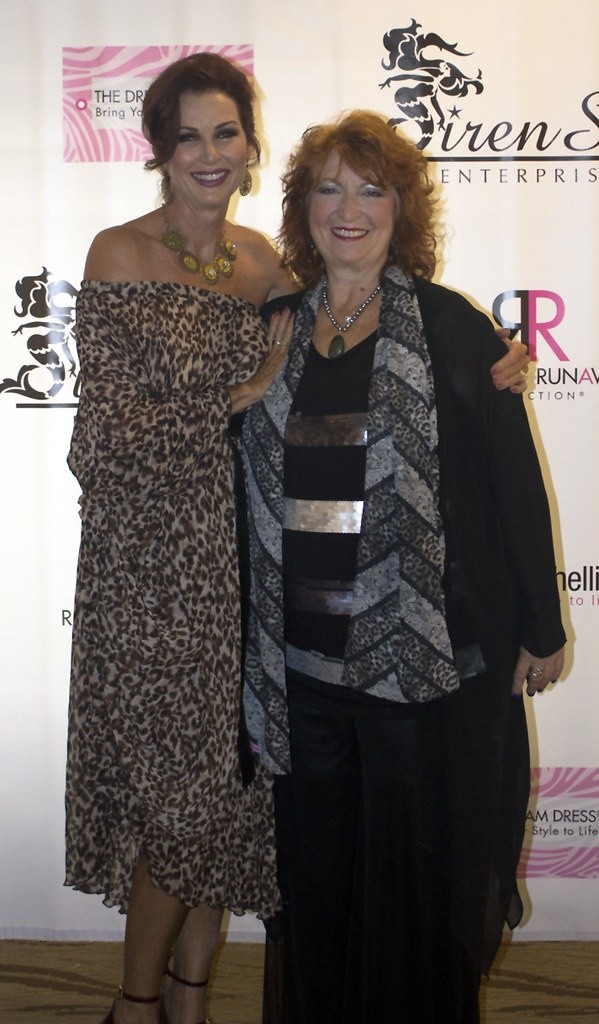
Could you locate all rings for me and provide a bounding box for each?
[528,668,544,678]
[520,370,528,380]
[273,339,288,347]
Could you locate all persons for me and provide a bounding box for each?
[62,56,302,1024]
[236,113,567,1024]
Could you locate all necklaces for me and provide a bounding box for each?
[321,274,384,360]
[162,203,237,284]
